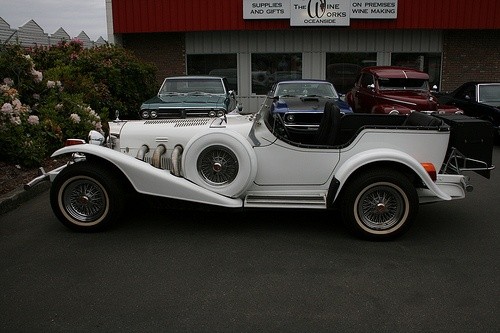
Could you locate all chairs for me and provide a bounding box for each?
[308,99,341,145]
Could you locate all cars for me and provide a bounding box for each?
[141,74,238,121]
[347,66,465,118]
[432,81,499,142]
[23,90,496,242]
[268,78,353,130]
